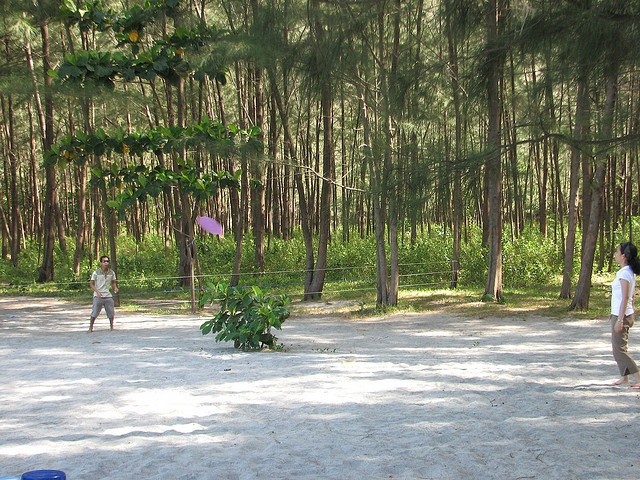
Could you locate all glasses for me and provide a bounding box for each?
[101,260,110,263]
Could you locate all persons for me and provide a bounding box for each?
[609,241,640,390]
[87,256,121,333]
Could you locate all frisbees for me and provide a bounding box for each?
[198,216,223,236]
